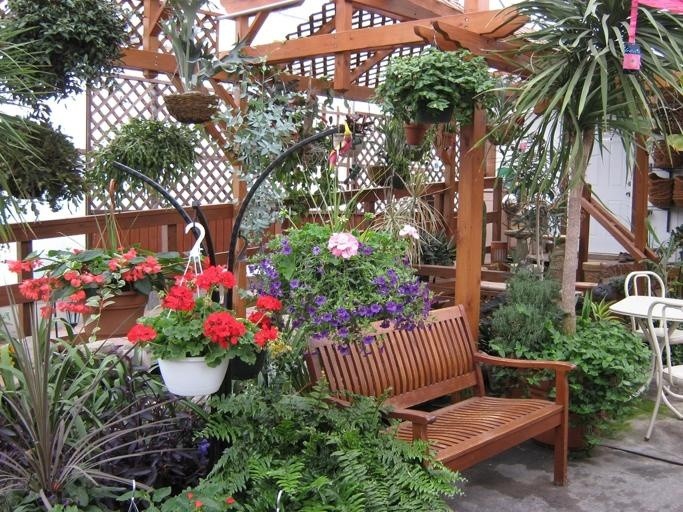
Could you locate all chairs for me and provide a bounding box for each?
[645,299,683,441]
[622,270,683,403]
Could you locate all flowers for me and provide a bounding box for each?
[6,240,177,318]
[127,252,282,367]
[236,120,447,360]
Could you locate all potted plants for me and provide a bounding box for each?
[479,293,654,459]
[0,0,331,200]
[369,0,683,243]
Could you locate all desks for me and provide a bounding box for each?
[609,295,683,400]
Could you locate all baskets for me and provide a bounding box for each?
[648,104,683,209]
[486,123,515,145]
[368,165,391,186]
[163,92,216,123]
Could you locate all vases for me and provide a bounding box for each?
[80,292,147,342]
[155,353,233,397]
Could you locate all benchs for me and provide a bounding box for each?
[301,304,577,487]
